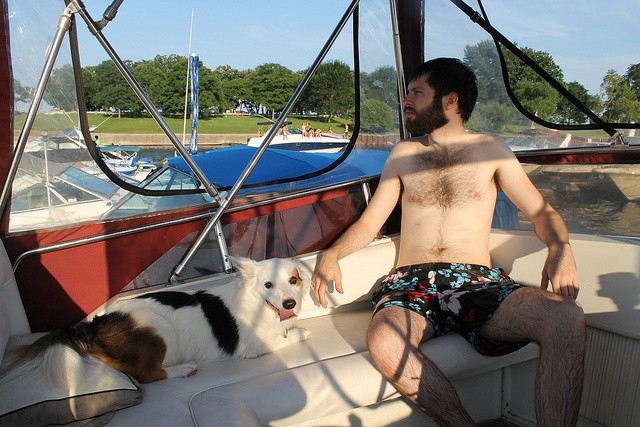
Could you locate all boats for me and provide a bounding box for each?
[22,128,140,174]
[8,155,213,232]
[592,164,640,203]
[99,144,391,220]
[540,121,640,201]
[505,122,592,193]
[248,129,349,153]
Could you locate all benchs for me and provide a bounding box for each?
[0,234,540,427]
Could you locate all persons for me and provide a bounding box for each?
[95,136,100,146]
[312,58,586,427]
[276,124,291,141]
[328,127,335,134]
[344,124,349,140]
[302,122,322,140]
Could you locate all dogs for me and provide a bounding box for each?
[0,254,317,394]
